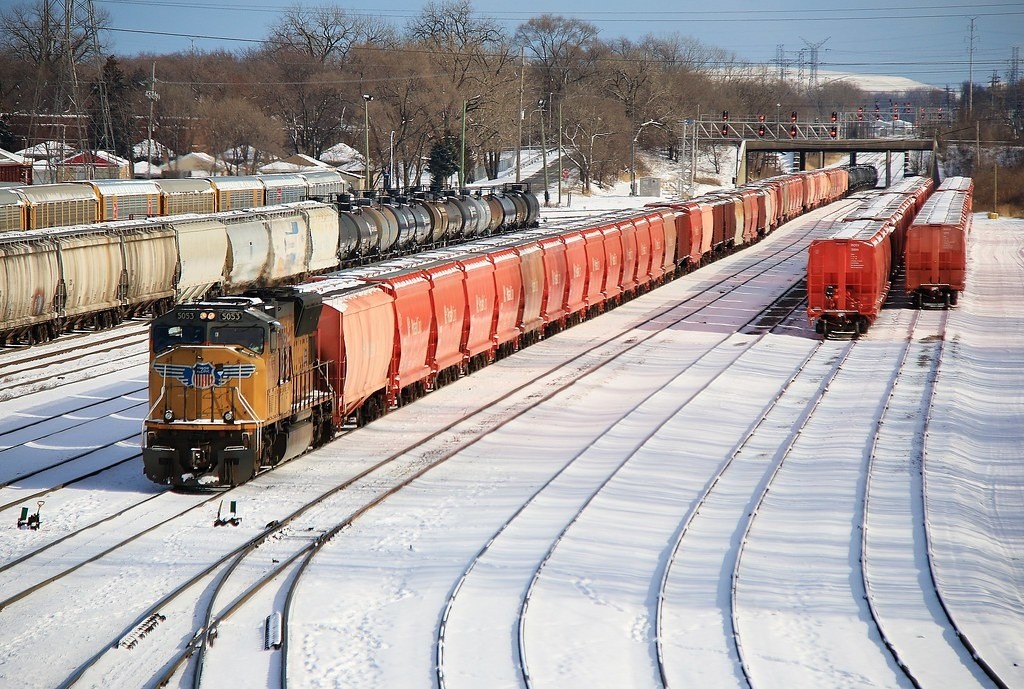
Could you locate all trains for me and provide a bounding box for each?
[904,176,975,310]
[1,179,540,354]
[1,169,347,239]
[803,175,937,340]
[143,163,878,494]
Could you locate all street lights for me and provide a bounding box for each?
[458,93,482,189]
[538,98,549,205]
[361,92,375,192]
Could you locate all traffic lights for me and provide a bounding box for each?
[721,101,944,139]
